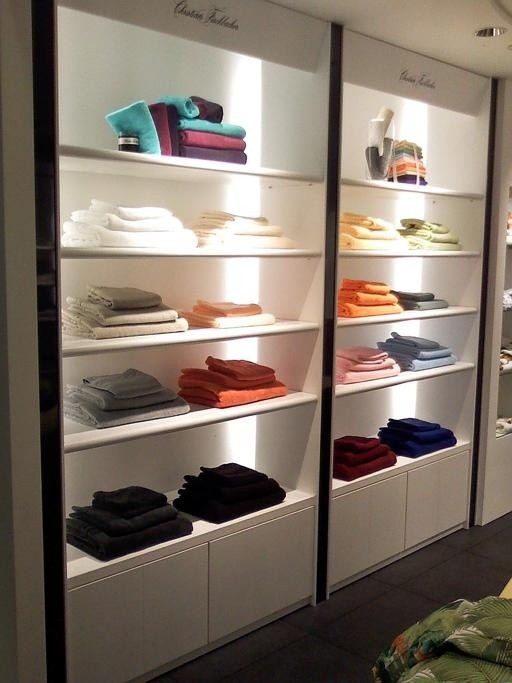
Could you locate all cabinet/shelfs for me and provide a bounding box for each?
[330,24,494,601]
[468,77,510,528]
[5,3,330,683]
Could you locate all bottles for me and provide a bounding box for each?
[117,129,139,151]
